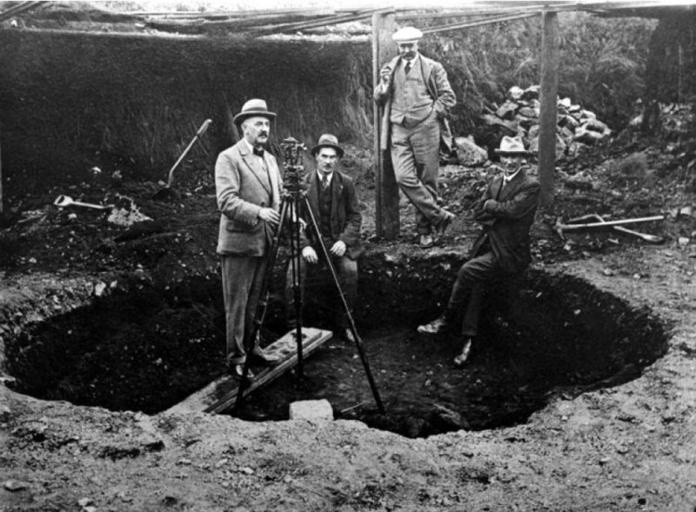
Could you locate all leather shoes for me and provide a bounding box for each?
[450,338,477,366]
[413,317,454,337]
[226,352,284,383]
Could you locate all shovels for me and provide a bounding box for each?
[151,118,212,197]
[569,214,660,243]
[54,194,130,215]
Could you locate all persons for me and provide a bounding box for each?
[212,98,284,382]
[283,132,362,346]
[372,26,456,249]
[414,134,541,368]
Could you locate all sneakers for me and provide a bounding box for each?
[417,210,457,250]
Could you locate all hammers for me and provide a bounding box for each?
[552,216,664,240]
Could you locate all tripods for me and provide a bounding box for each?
[232,182,387,416]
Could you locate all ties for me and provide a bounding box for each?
[319,172,330,191]
[402,59,413,75]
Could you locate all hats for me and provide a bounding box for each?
[492,133,534,158]
[388,25,423,44]
[309,134,344,158]
[228,97,278,124]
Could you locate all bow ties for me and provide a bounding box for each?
[251,148,266,156]
